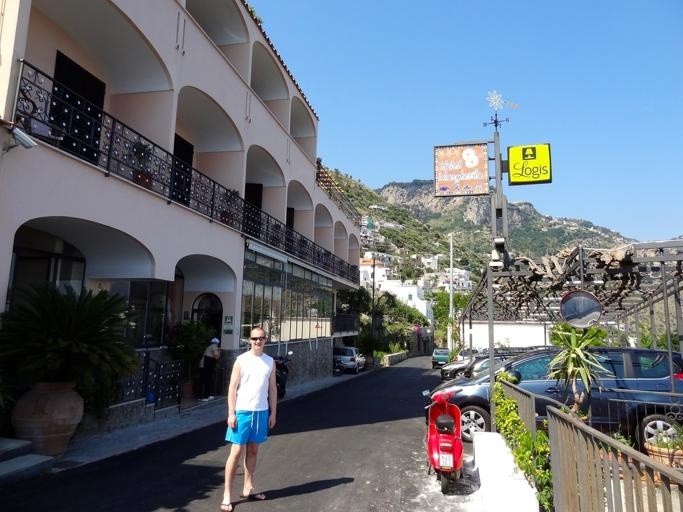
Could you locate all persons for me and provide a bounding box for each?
[196,337,220,404]
[219,326,279,512]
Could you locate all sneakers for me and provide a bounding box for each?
[197,395,215,402]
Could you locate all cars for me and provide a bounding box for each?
[431,348,449,369]
[333,345,367,375]
[457,347,478,362]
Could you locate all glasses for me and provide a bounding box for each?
[251,336,266,340]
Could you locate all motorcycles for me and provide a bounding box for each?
[421,385,464,496]
[334,359,345,376]
[270,350,294,398]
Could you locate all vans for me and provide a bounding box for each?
[425,344,681,452]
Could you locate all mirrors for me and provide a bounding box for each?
[559,289,602,330]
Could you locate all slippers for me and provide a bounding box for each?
[240,491,266,500]
[221,500,233,512]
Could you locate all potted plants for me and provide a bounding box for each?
[644,424,683,484]
[133,141,153,189]
[298,239,359,283]
[0,278,140,465]
[221,188,240,226]
[168,319,216,398]
[271,223,281,248]
[600,432,640,479]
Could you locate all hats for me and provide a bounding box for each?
[210,338,220,344]
